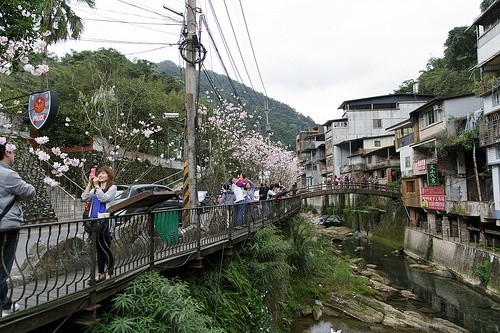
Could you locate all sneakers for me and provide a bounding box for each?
[2,303,19,317]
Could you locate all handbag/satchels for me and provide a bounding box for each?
[83,189,97,219]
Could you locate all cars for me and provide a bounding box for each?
[198,190,212,213]
[112,184,183,226]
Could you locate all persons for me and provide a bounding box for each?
[222,183,236,230]
[230,177,245,227]
[363,177,368,188]
[81,167,117,282]
[322,175,327,190]
[242,179,256,227]
[327,176,331,188]
[334,175,340,185]
[259,180,282,219]
[290,185,296,194]
[345,177,349,188]
[0,143,36,314]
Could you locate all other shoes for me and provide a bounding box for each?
[233,226,242,229]
[88,275,106,282]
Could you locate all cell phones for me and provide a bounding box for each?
[91,167,97,176]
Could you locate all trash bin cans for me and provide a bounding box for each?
[154,208,180,247]
